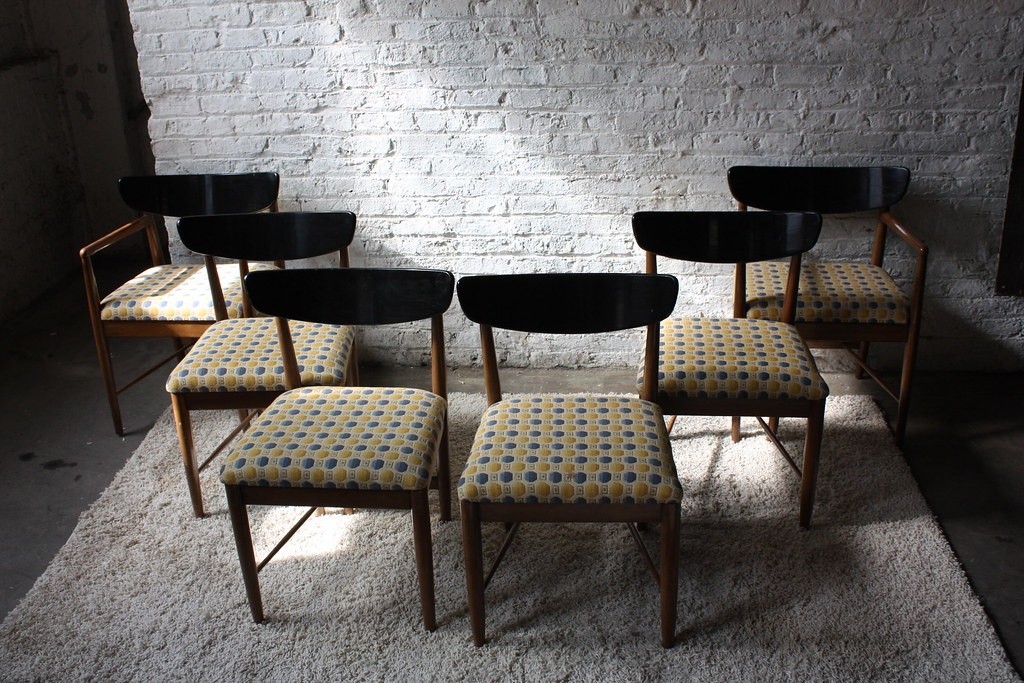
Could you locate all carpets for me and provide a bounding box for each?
[0,393,1024,683]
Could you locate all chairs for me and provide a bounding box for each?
[458,275,684,647]
[727,165,928,447]
[633,211,829,527]
[219,267,451,629]
[80,173,279,434]
[166,210,358,516]
